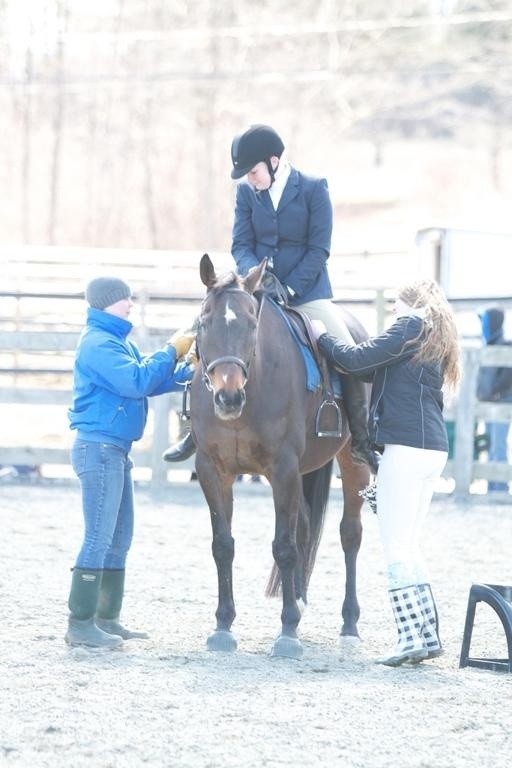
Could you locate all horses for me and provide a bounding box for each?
[189,252,376,660]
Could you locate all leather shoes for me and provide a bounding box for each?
[163,433,196,462]
[351,442,381,474]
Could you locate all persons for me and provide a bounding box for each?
[162,124,379,475]
[475,308,511,492]
[62,277,198,651]
[310,277,462,667]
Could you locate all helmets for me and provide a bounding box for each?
[231,125,283,179]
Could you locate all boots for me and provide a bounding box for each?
[375,584,442,666]
[67,568,149,648]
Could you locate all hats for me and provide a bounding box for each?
[87,277,131,309]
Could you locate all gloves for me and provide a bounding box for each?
[311,319,326,338]
[168,329,197,357]
